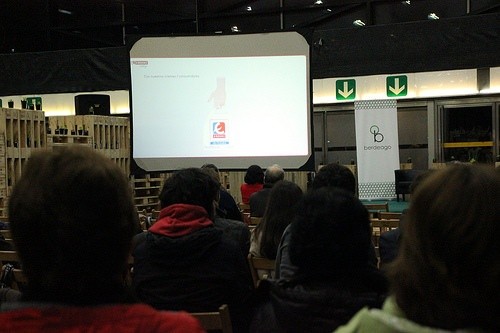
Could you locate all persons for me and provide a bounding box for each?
[1,144,500,332]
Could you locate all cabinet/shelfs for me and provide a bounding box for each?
[0,109,166,217]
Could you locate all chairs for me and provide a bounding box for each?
[0,169,416,333]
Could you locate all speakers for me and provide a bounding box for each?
[75,94,110,115]
